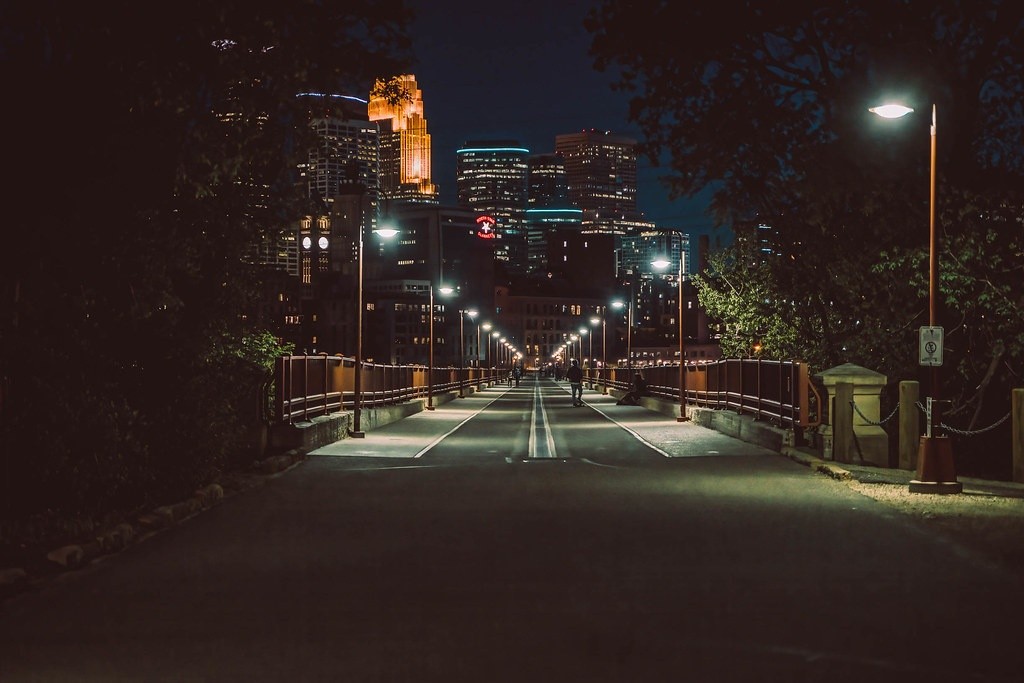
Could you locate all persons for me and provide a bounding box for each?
[553,362,570,380]
[549,366,553,377]
[545,369,547,376]
[616,373,647,405]
[514,367,521,386]
[539,368,542,377]
[566,359,583,407]
[506,369,513,387]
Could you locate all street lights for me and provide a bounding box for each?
[350,188,403,441]
[487,330,501,388]
[562,345,568,381]
[422,283,455,410]
[612,299,634,394]
[494,337,506,384]
[566,340,576,359]
[578,327,593,391]
[456,305,480,398]
[869,94,966,495]
[475,322,491,392]
[572,335,583,367]
[504,342,517,374]
[590,316,608,395]
[650,228,690,424]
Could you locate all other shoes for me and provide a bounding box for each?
[573,404,577,408]
[577,397,583,403]
